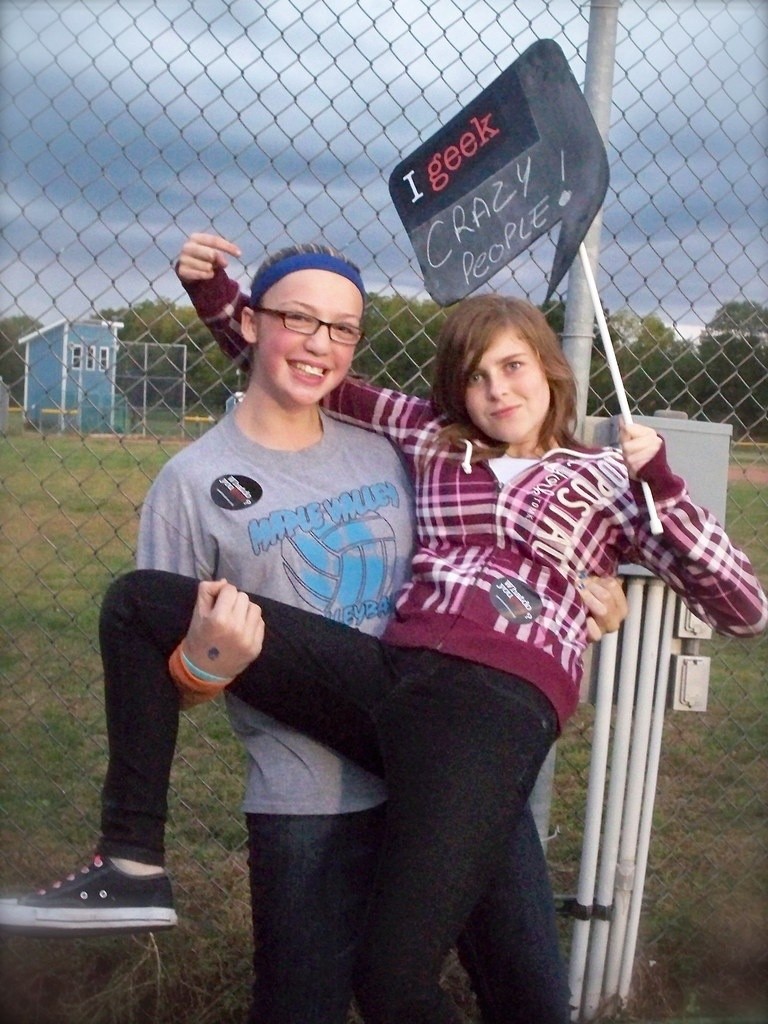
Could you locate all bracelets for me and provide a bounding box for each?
[183,652,227,683]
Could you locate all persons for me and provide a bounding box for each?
[1,232,768,1024]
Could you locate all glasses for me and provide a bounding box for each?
[253,305,365,345]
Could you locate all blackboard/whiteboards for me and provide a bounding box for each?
[388,38,611,312]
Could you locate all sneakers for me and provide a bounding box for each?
[0,855,179,936]
